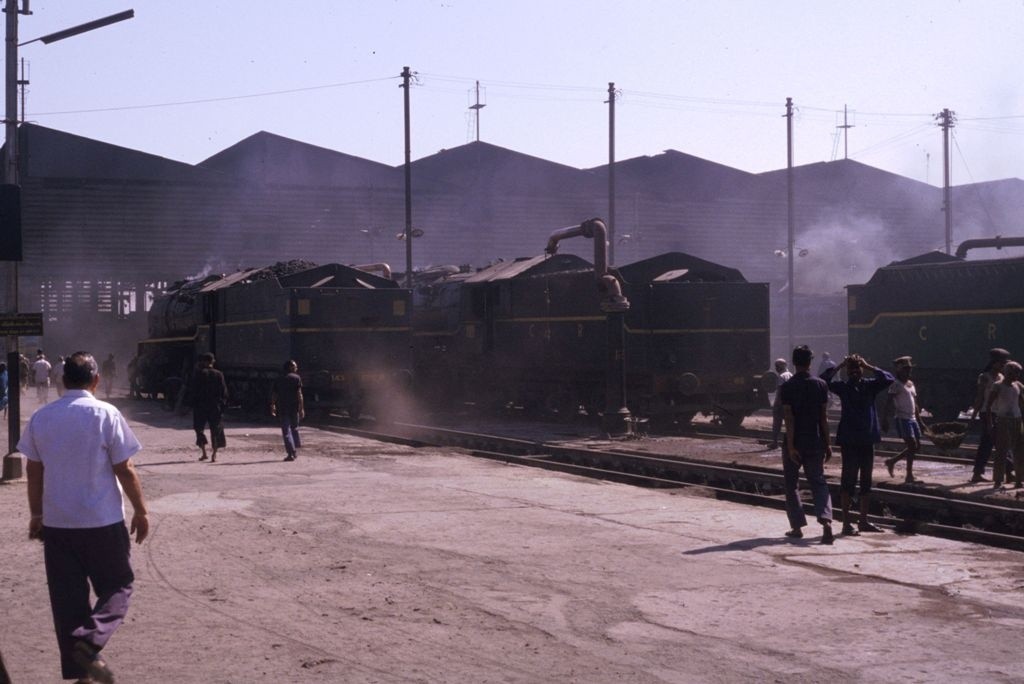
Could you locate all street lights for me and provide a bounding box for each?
[5,9,134,453]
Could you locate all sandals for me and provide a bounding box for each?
[70,639,115,684]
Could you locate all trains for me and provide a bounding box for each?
[137,236,1023,437]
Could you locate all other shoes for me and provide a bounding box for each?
[294,455,297,458]
[284,456,294,461]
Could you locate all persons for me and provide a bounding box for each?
[768,344,1024,545]
[0,350,141,419]
[16,353,149,684]
[192,352,228,463]
[272,360,304,461]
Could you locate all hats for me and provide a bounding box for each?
[990,348,1011,362]
[892,356,912,368]
[776,359,787,369]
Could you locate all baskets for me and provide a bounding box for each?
[923,422,968,449]
